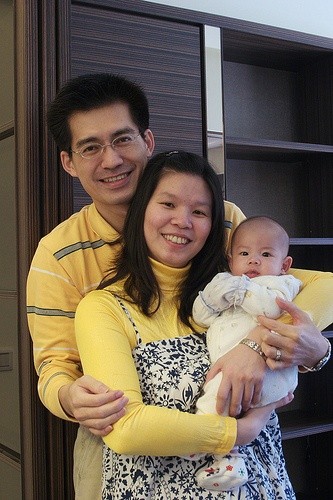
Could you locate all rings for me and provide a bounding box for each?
[276,349,281,361]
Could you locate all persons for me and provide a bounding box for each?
[73,151,333,500]
[189,216,306,493]
[23,73,332,500]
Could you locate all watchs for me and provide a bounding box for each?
[302,336,331,373]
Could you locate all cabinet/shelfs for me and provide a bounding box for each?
[13,0,333,500]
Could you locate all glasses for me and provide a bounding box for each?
[70,131,142,160]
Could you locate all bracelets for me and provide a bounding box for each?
[239,339,268,361]
